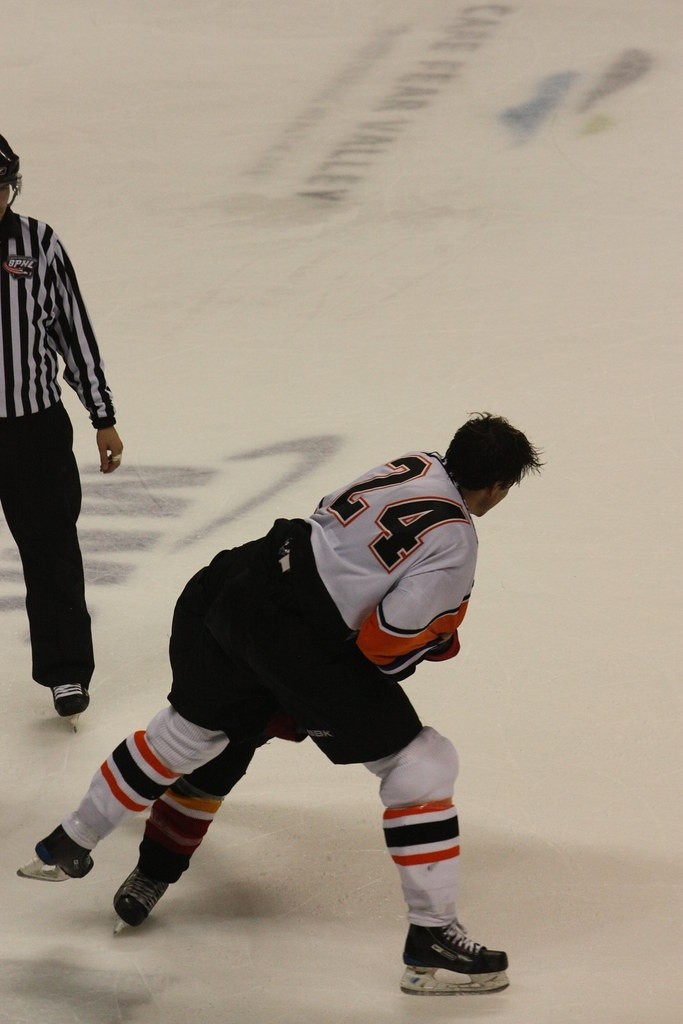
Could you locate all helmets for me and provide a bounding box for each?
[0,134,20,184]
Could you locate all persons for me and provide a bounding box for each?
[18,411,538,994]
[113,623,459,937]
[0,133,124,730]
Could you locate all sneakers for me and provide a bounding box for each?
[50,683,90,733]
[113,865,169,935]
[400,917,509,996]
[16,824,94,882]
